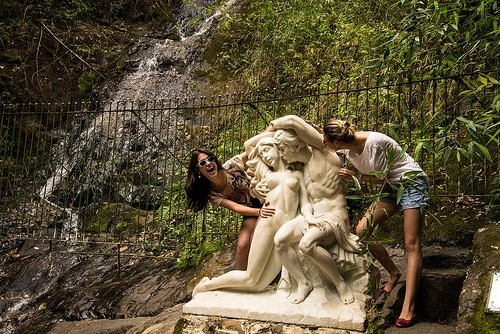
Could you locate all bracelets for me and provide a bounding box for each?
[358,174,362,181]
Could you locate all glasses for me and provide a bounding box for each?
[196,155,217,168]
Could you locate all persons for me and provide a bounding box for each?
[187,124,275,271]
[305,120,428,328]
[192,114,354,304]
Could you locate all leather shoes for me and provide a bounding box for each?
[395,310,418,327]
[382,271,402,296]
